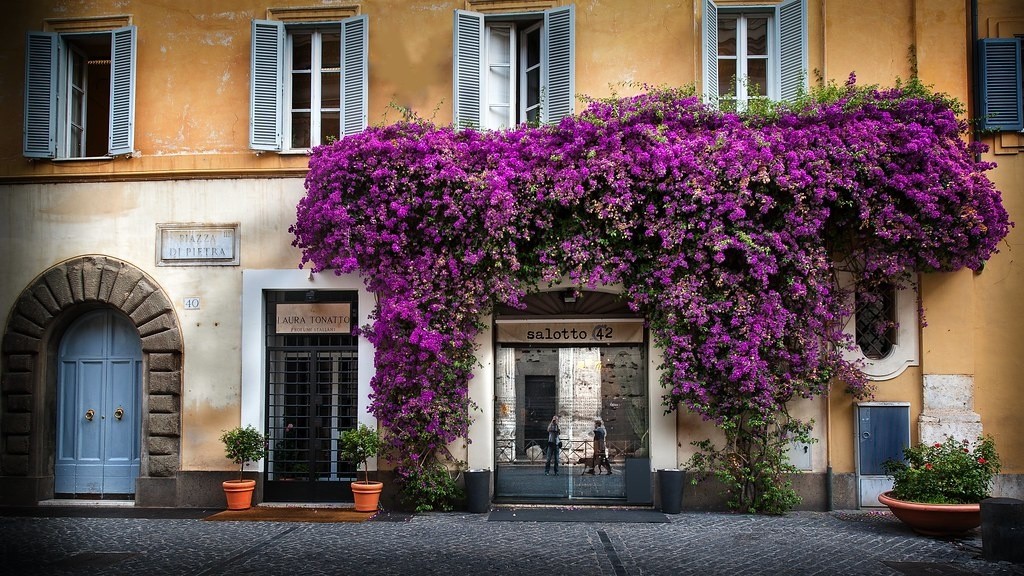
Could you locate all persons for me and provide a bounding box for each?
[593,420,612,475]
[545,416,560,476]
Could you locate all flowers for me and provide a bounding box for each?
[218,423,267,465]
[879,432,1001,504]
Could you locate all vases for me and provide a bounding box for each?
[877,490,999,535]
[222,479,256,510]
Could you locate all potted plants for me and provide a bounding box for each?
[339,423,385,512]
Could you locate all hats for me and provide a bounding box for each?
[552,415,559,421]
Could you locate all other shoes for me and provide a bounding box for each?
[585,470,595,475]
[605,472,612,476]
[544,472,550,477]
[554,473,560,476]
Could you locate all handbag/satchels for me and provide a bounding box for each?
[558,441,562,448]
